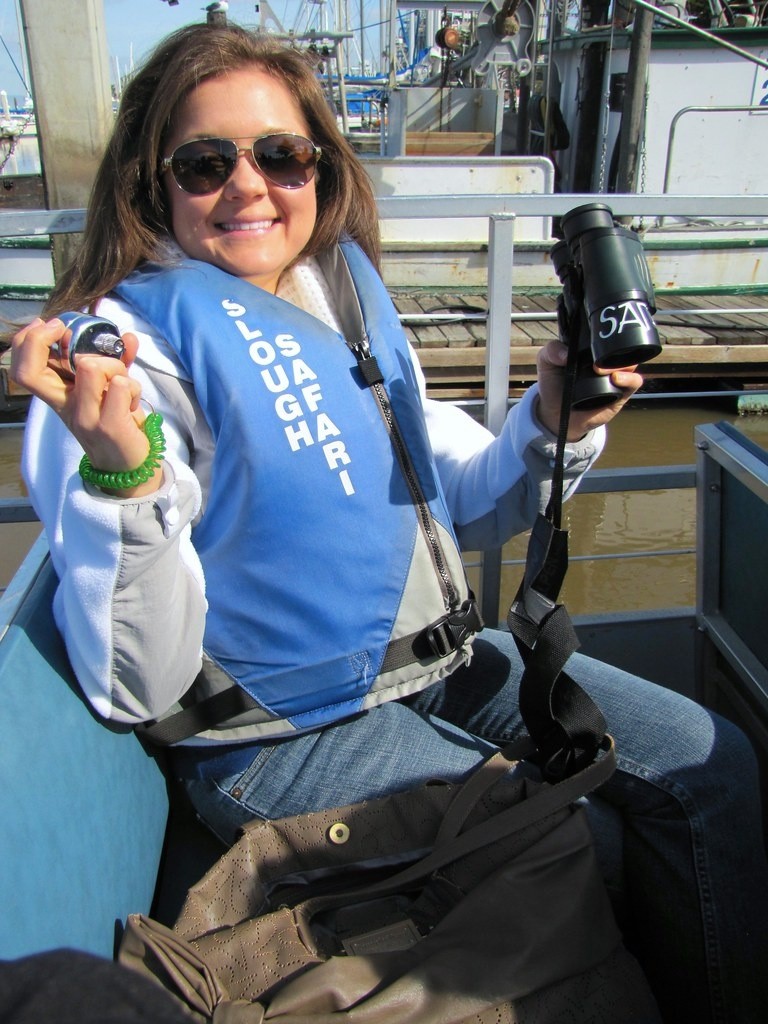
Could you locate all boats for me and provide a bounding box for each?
[1,0,768,297]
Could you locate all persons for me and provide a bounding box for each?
[11,24,762,1012]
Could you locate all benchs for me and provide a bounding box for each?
[0,528,222,1024]
[694,421,768,754]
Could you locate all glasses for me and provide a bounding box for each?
[160,133,322,196]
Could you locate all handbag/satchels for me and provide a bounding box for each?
[118,732,662,1024]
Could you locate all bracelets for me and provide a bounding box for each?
[73,408,167,490]
